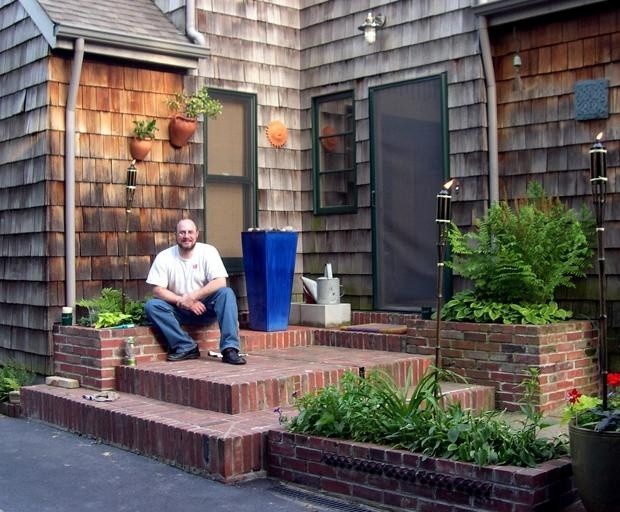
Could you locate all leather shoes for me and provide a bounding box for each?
[167,344,200,361]
[222,350,247,364]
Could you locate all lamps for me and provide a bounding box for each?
[358,11,386,45]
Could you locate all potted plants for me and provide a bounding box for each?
[132,118,159,160]
[167,88,223,148]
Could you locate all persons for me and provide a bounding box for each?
[145,217,247,364]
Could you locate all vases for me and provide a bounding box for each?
[569,410,620,512]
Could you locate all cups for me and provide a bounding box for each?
[61,312,73,327]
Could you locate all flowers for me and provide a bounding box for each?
[558,373,620,428]
